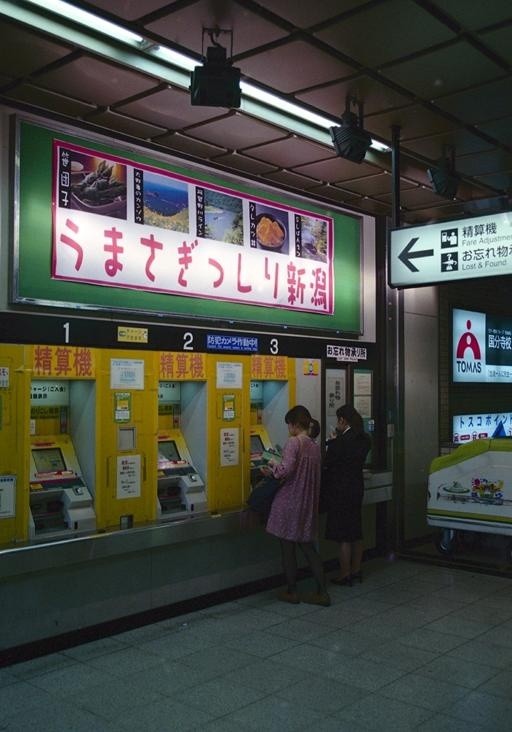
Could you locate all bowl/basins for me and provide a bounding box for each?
[253,213,288,252]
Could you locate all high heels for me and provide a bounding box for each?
[331,570,361,587]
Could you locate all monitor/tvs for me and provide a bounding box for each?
[158,440,181,464]
[250,434,264,454]
[31,447,67,474]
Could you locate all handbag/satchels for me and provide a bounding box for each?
[247,475,286,512]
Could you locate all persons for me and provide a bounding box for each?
[264,406,330,607]
[324,405,369,586]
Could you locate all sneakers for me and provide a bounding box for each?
[303,592,331,607]
[277,592,300,604]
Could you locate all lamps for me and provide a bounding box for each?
[424,135,463,202]
[187,23,244,112]
[327,93,375,166]
